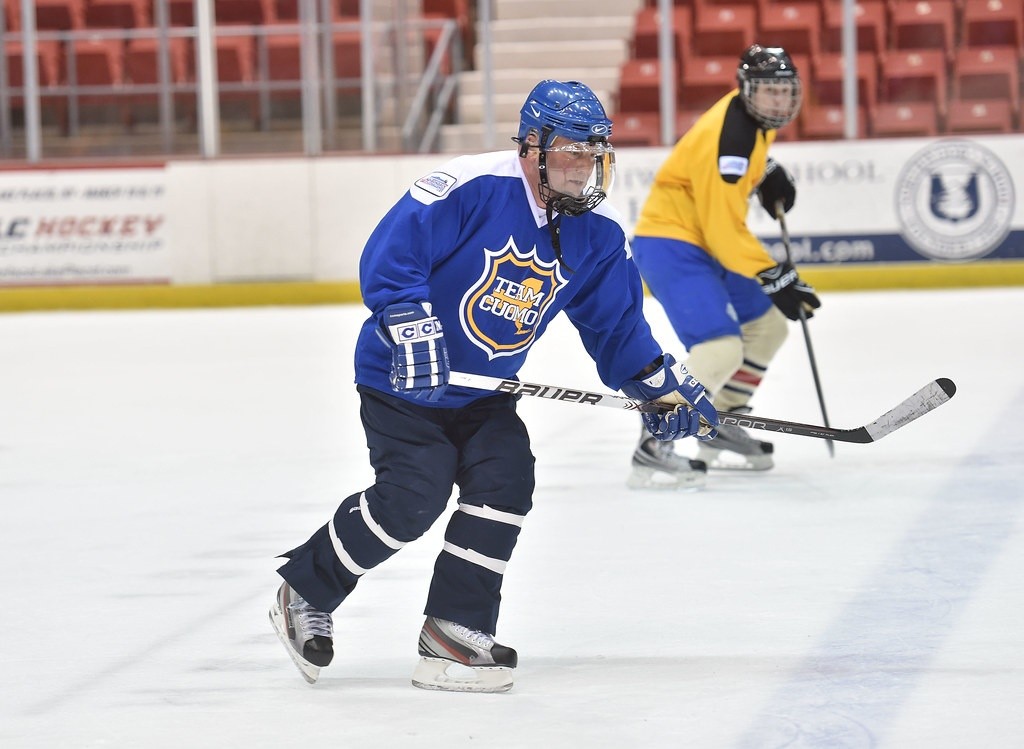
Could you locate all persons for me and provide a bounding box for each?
[268,79,716,694]
[629,44,822,472]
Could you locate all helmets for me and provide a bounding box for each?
[517,80,614,156]
[735,43,798,101]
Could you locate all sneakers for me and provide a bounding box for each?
[696,405,774,471]
[412,615,517,693]
[622,423,707,489]
[268,580,334,685]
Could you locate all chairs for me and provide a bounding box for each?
[0,0,1024,145]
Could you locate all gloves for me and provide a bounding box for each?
[375,300,449,401]
[757,156,796,219]
[620,353,720,441]
[756,260,822,321]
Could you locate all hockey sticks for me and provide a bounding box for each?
[448,369,958,445]
[776,199,836,457]
